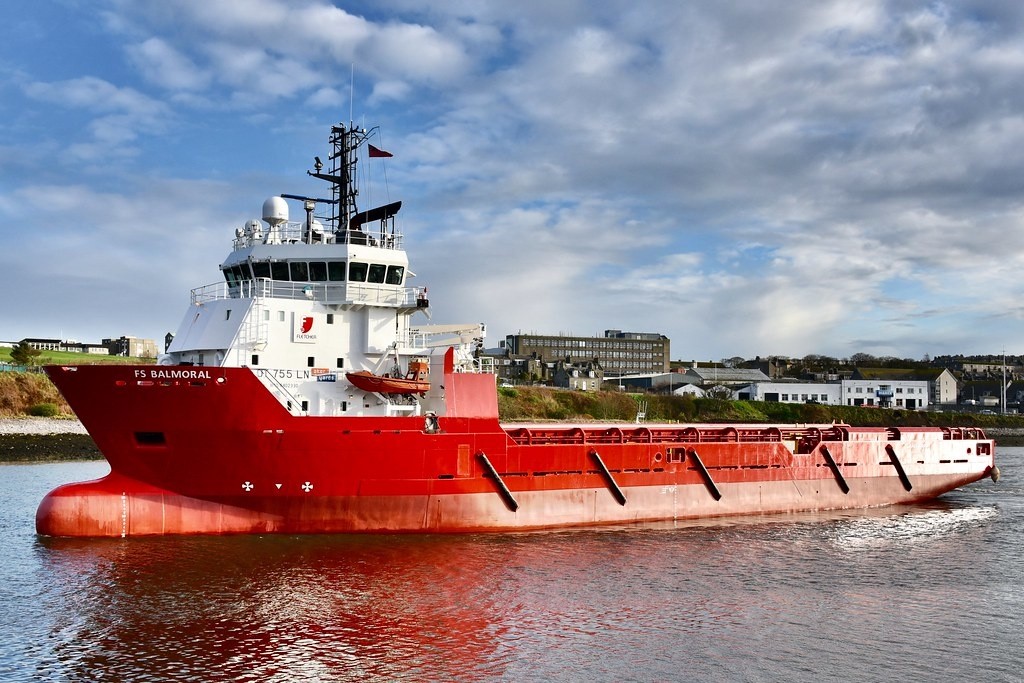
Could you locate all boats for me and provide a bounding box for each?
[34,59,1001,540]
[345,362,431,399]
